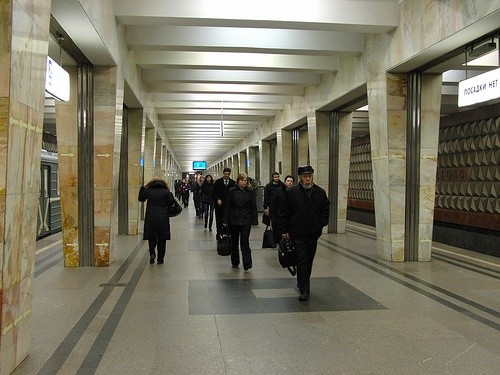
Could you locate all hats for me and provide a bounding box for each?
[298,165,314,175]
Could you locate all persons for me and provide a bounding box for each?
[217,172,258,271]
[138,176,182,264]
[174,168,257,232]
[278,165,330,302]
[262,172,295,248]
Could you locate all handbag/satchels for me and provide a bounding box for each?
[216,228,233,256]
[167,192,182,217]
[262,212,270,225]
[278,237,297,268]
[263,225,276,248]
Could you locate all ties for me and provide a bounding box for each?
[225,181,228,185]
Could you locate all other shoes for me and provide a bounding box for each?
[232,264,238,268]
[299,289,309,301]
[158,259,164,264]
[150,254,155,264]
[244,262,252,270]
[205,223,207,228]
[209,226,212,231]
